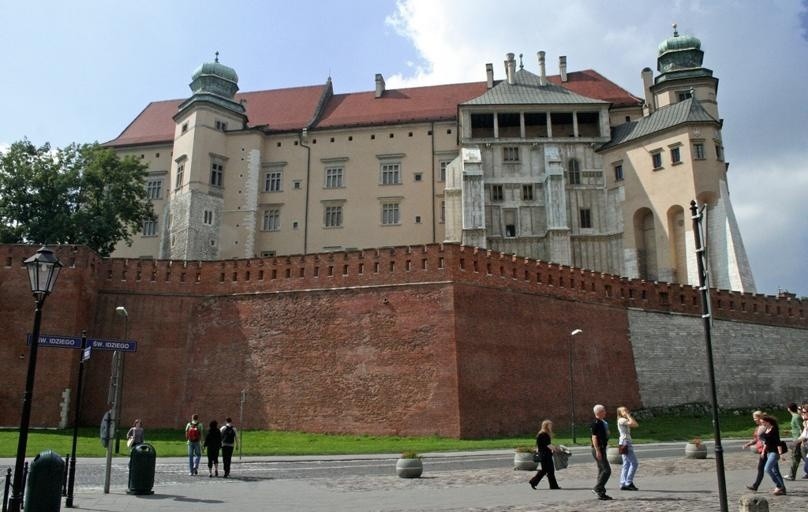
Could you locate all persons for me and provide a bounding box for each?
[204,421,222,477]
[743,402,808,496]
[590,405,614,500]
[528,420,560,489]
[617,407,640,490]
[219,417,239,478]
[127,418,144,465]
[185,414,204,476]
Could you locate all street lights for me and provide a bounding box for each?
[569,326,584,442]
[116,304,129,434]
[4,240,67,512]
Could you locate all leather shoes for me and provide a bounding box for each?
[529,481,536,490]
[747,485,757,491]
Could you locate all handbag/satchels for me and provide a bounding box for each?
[618,445,629,454]
[777,440,789,455]
[533,452,544,462]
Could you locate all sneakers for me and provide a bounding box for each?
[592,488,605,499]
[625,483,638,490]
[782,475,794,480]
[599,495,612,500]
[771,488,786,495]
[801,472,808,479]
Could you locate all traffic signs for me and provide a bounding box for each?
[86,337,139,353]
[24,334,82,350]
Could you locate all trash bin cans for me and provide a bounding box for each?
[23,449,65,512]
[125,443,156,495]
